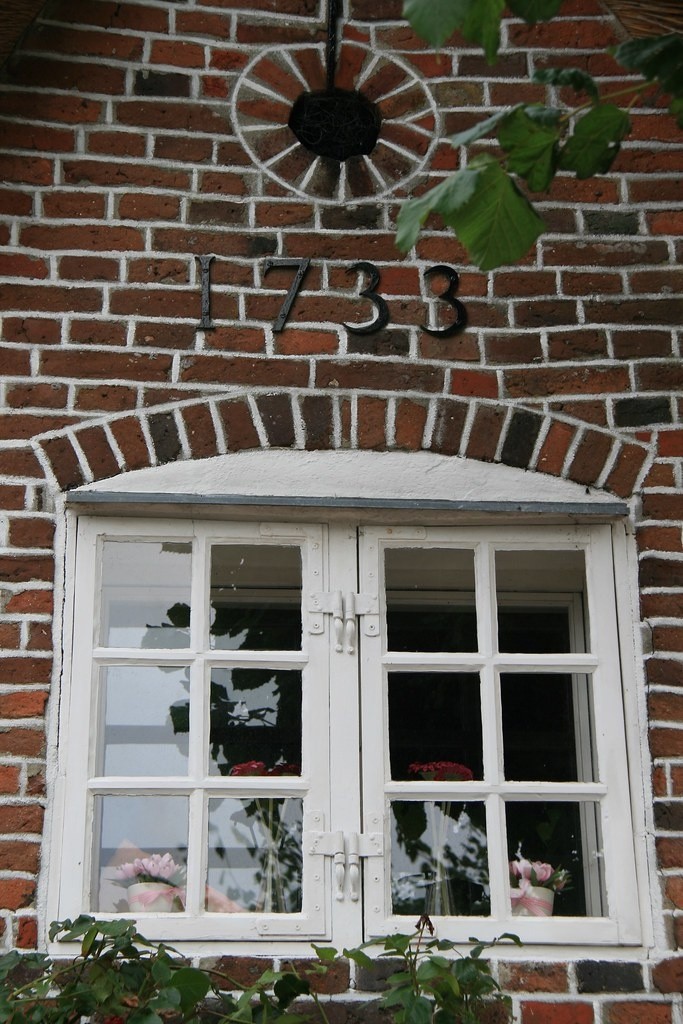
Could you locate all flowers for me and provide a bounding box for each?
[408,761,473,915]
[228,761,299,911]
[106,852,188,886]
[510,858,575,894]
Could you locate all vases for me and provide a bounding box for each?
[251,806,291,912]
[126,881,173,912]
[421,843,458,916]
[511,886,556,917]
